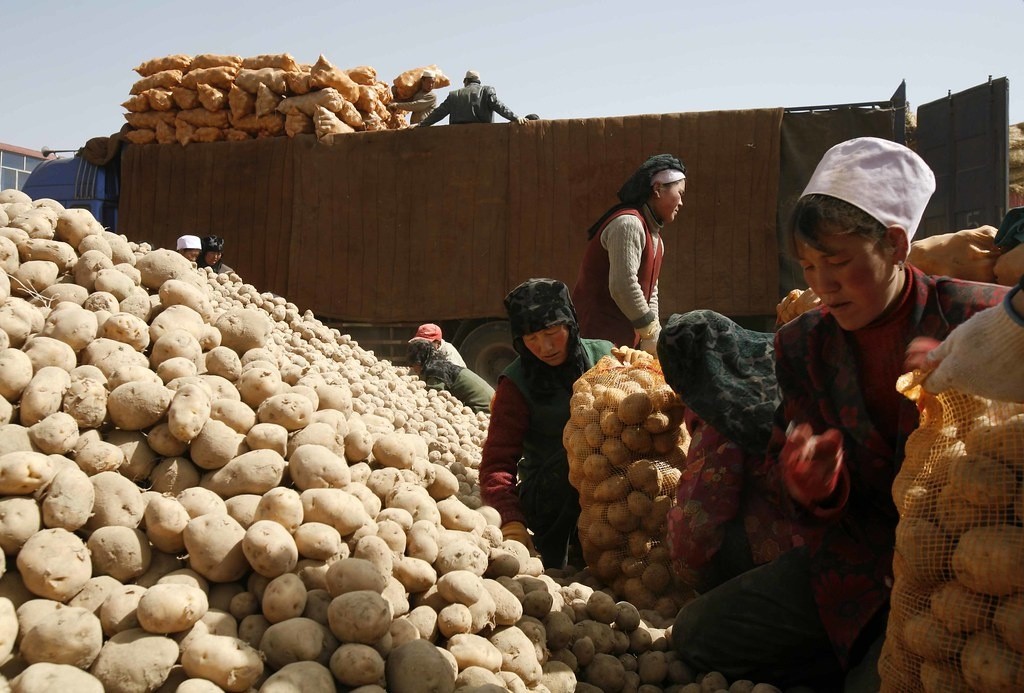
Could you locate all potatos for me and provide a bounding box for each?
[0,54,1024,693]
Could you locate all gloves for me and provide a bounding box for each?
[784,423,842,500]
[921,284,1024,403]
[504,522,538,557]
[639,325,661,357]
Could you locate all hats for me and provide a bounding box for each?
[176,235,202,251]
[798,136,938,245]
[422,70,436,78]
[651,169,686,186]
[464,70,479,82]
[408,323,443,346]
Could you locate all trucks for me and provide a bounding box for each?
[19,75,1011,391]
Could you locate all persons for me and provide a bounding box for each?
[388,71,436,124]
[479,278,616,572]
[197,235,233,274]
[408,324,467,368]
[572,155,686,358]
[407,70,528,133]
[657,137,1023,693]
[525,114,539,120]
[407,339,495,413]
[177,235,202,261]
